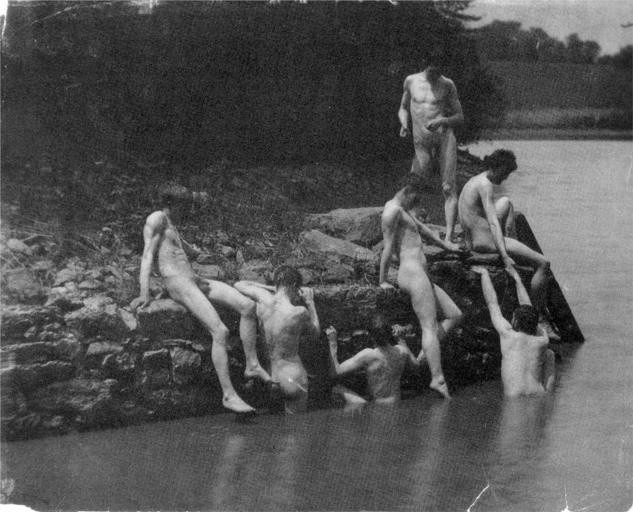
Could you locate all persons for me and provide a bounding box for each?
[231,266,320,414]
[128,185,282,414]
[324,313,419,409]
[455,148,562,341]
[468,263,559,400]
[377,170,471,400]
[396,50,464,250]
